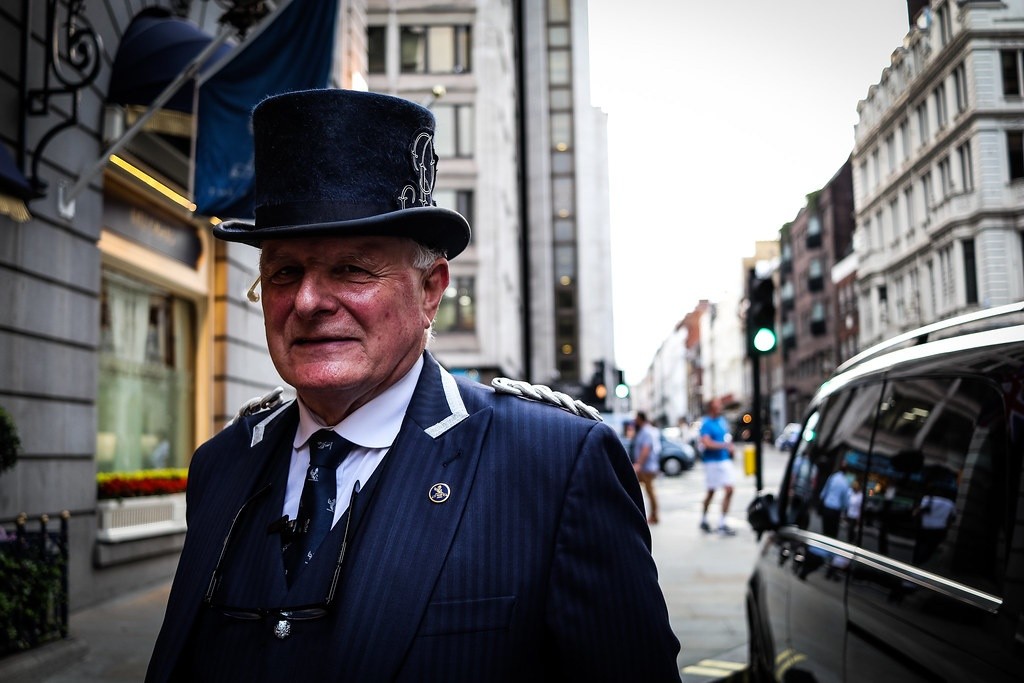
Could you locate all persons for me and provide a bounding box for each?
[789,445,958,582]
[631,409,658,524]
[700,399,735,534]
[144,89,681,683]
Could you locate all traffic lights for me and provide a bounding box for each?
[748,268,777,356]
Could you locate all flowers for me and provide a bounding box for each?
[95,467,195,497]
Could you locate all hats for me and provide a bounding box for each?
[206,89,472,260]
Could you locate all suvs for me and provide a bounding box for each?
[744,299,1024,682]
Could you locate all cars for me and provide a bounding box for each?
[658,432,699,477]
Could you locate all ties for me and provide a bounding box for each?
[284,428,360,590]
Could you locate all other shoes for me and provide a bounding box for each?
[701,523,735,535]
